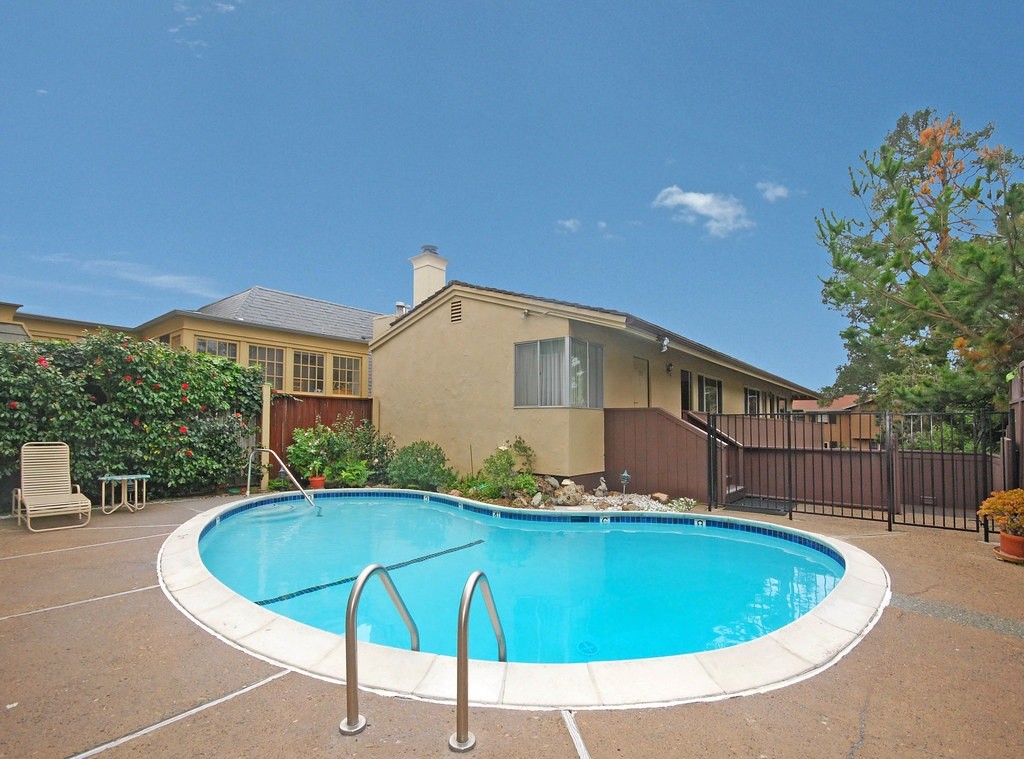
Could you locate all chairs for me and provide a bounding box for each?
[11,441,92,532]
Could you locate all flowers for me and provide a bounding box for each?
[289,427,330,477]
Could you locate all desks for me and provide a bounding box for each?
[98,475,151,514]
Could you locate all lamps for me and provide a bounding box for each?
[619,469,631,493]
[657,334,670,353]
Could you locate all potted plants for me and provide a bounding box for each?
[977,489,1024,557]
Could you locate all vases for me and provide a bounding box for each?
[308,477,326,488]
